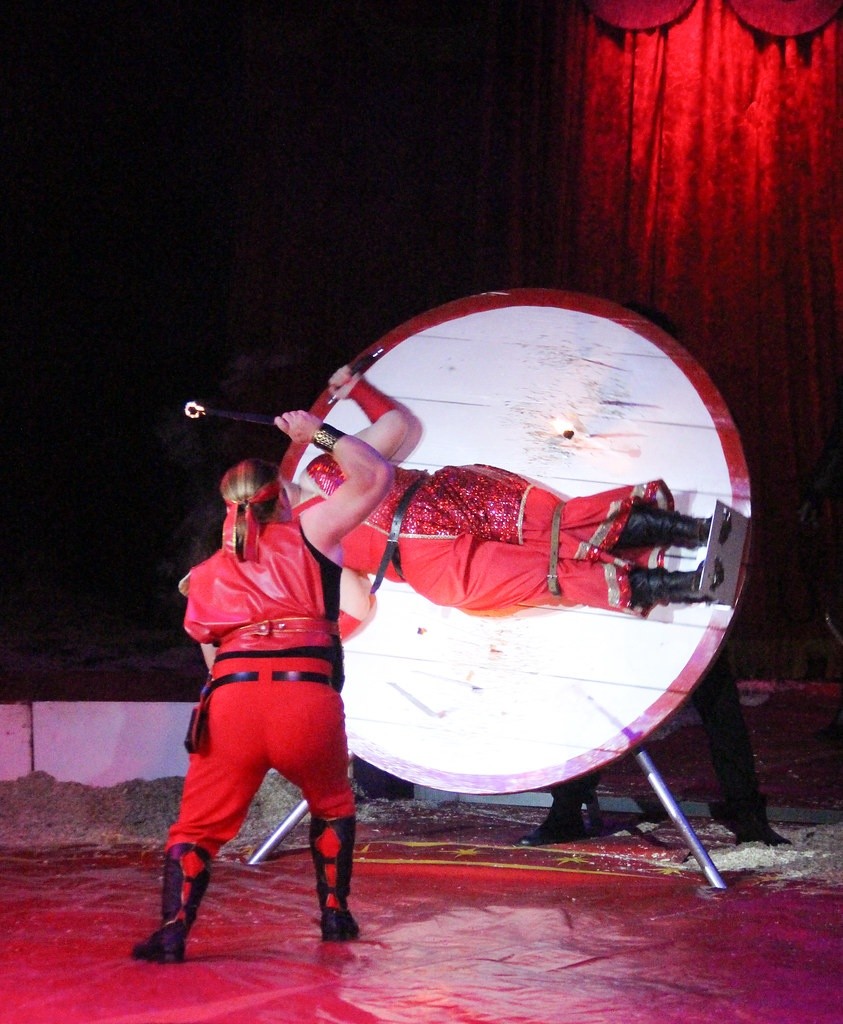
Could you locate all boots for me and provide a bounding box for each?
[617,498,729,550]
[629,558,724,609]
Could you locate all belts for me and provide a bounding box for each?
[370,475,424,593]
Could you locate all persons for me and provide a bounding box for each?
[130,410,395,961]
[277,364,734,619]
[519,301,791,847]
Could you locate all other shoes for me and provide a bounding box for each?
[321,907,359,941]
[518,790,604,847]
[132,923,184,962]
[734,814,790,847]
